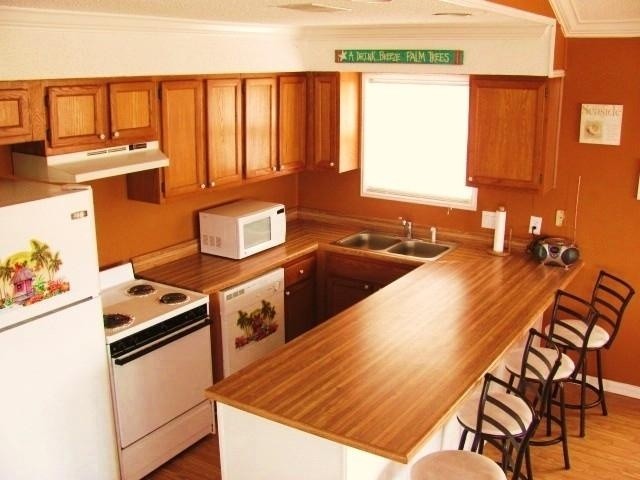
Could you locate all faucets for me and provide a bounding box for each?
[399,216,413,241]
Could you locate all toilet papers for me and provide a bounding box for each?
[493,210,506,253]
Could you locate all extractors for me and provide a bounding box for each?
[11,139,171,186]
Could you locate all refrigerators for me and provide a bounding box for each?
[0,177,125,479]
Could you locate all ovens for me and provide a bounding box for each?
[109,303,215,480]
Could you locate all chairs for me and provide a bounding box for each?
[545,270,635,436]
[505,290,600,470]
[458,328,561,480]
[409,371,539,480]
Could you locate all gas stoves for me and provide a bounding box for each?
[99,280,210,342]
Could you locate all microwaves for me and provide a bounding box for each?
[199,196,289,261]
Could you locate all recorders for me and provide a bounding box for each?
[529,236,580,267]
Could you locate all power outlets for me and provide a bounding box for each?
[529,215,544,235]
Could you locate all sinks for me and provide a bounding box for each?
[330,227,401,250]
[385,241,462,262]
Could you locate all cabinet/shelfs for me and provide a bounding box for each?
[308,72,359,177]
[242,72,309,185]
[285,250,317,341]
[466,76,562,198]
[11,77,160,157]
[0,81,44,147]
[318,247,417,325]
[127,74,243,206]
